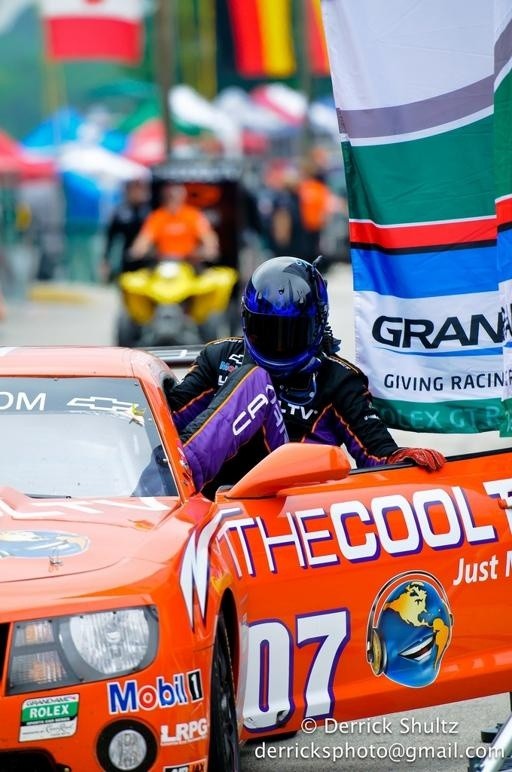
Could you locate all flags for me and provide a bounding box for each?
[42,1,149,64]
[228,0,327,81]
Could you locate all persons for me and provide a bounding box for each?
[165,254,445,497]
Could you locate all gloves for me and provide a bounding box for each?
[383,445,448,472]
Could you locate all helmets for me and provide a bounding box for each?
[239,253,342,382]
